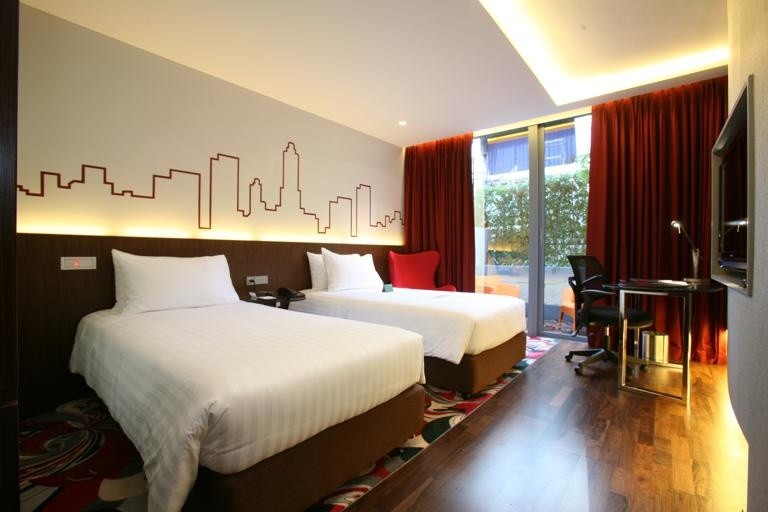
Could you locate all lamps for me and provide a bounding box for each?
[670,221,709,284]
[720,218,747,238]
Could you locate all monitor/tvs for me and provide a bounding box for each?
[717,122,746,288]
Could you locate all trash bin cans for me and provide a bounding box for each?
[642,331,670,364]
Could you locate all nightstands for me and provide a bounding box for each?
[243,296,289,309]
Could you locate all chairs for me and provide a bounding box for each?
[558,287,584,334]
[476,275,521,299]
[387,250,457,291]
[566,255,654,383]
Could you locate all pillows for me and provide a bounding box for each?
[111,249,239,314]
[321,247,384,292]
[306,251,361,291]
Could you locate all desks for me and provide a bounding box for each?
[719,251,747,274]
[617,279,723,408]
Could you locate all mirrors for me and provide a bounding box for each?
[710,74,755,298]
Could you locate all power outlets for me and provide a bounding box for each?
[247,275,269,286]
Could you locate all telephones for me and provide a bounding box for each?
[277,287,305,300]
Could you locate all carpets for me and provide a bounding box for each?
[18,337,562,512]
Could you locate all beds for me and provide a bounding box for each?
[82,300,425,512]
[290,287,526,397]
